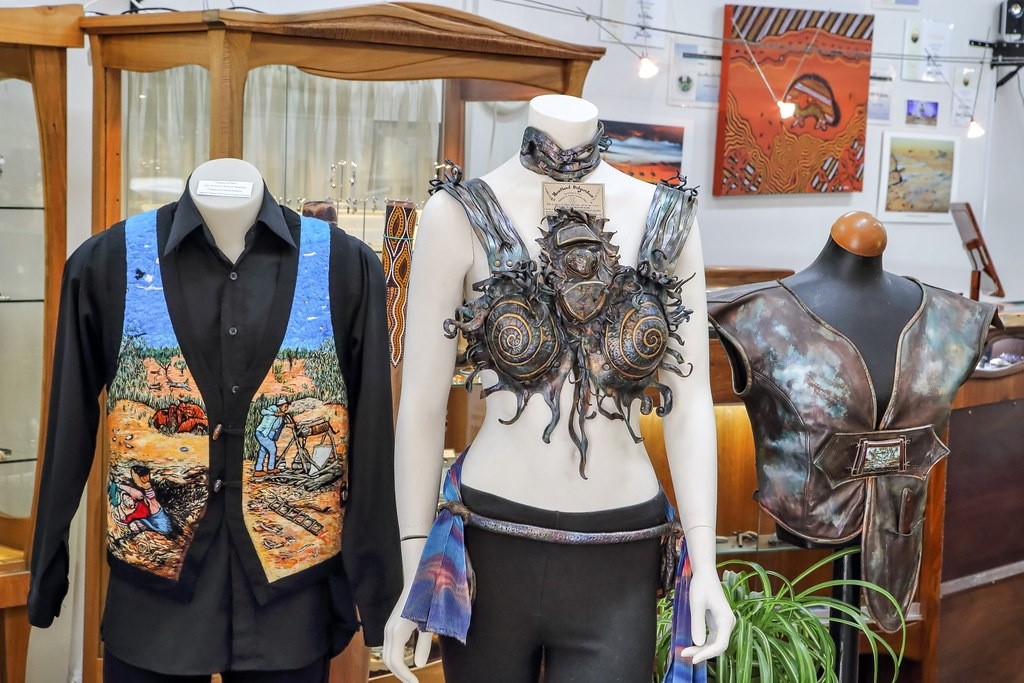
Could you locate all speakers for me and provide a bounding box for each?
[998,0,1024,42]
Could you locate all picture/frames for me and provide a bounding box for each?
[877,131,961,225]
[865,59,897,125]
[667,34,722,110]
[902,17,954,83]
[871,0,921,11]
[950,62,983,126]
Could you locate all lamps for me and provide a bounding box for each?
[730,10,835,121]
[576,1,659,78]
[921,26,992,139]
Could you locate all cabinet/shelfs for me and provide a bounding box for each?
[74,1,608,683]
[443,267,950,683]
[0,3,85,682]
